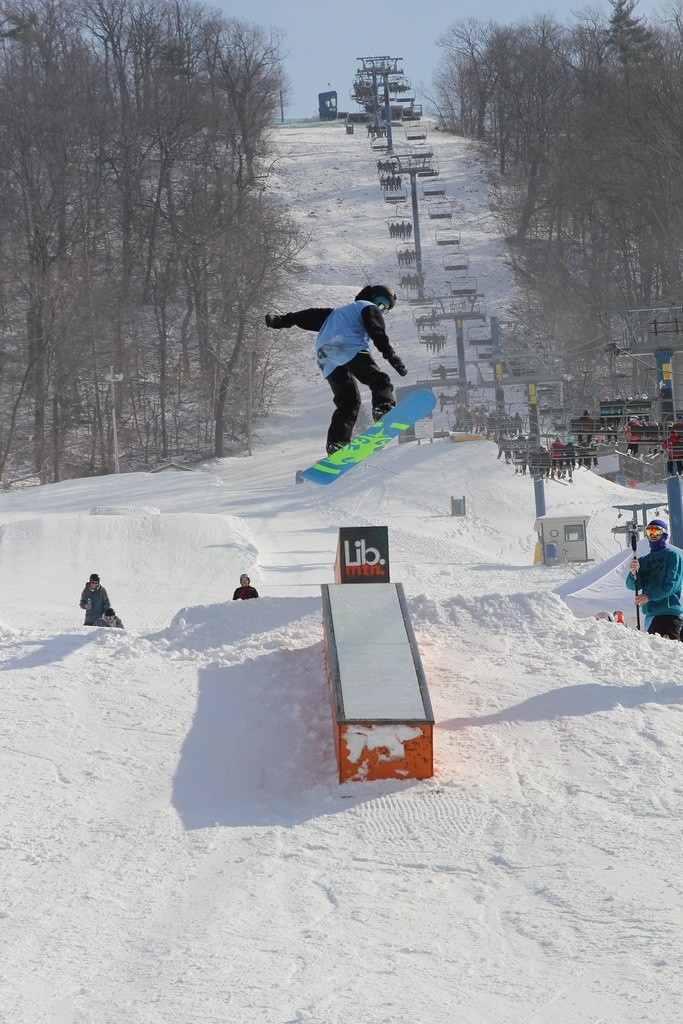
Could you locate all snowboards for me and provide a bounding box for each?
[296,388,437,485]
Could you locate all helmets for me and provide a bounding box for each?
[584,409,590,416]
[240,574,250,584]
[631,415,638,422]
[369,286,395,314]
[671,430,676,437]
[567,437,573,443]
[104,608,115,617]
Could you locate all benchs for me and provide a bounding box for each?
[349,48,682,486]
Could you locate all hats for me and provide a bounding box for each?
[555,436,560,441]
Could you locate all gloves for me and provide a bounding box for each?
[266,314,292,328]
[392,355,408,375]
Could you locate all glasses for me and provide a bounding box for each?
[645,526,668,536]
[378,303,389,314]
[89,574,99,585]
[106,616,113,620]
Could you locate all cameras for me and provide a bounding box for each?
[629,524,638,532]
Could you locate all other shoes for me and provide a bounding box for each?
[567,479,573,484]
[561,475,566,479]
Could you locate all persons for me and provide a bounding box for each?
[233,573,259,601]
[594,610,625,624]
[438,389,683,483]
[93,607,124,629]
[626,519,683,643]
[265,284,408,459]
[80,574,111,627]
